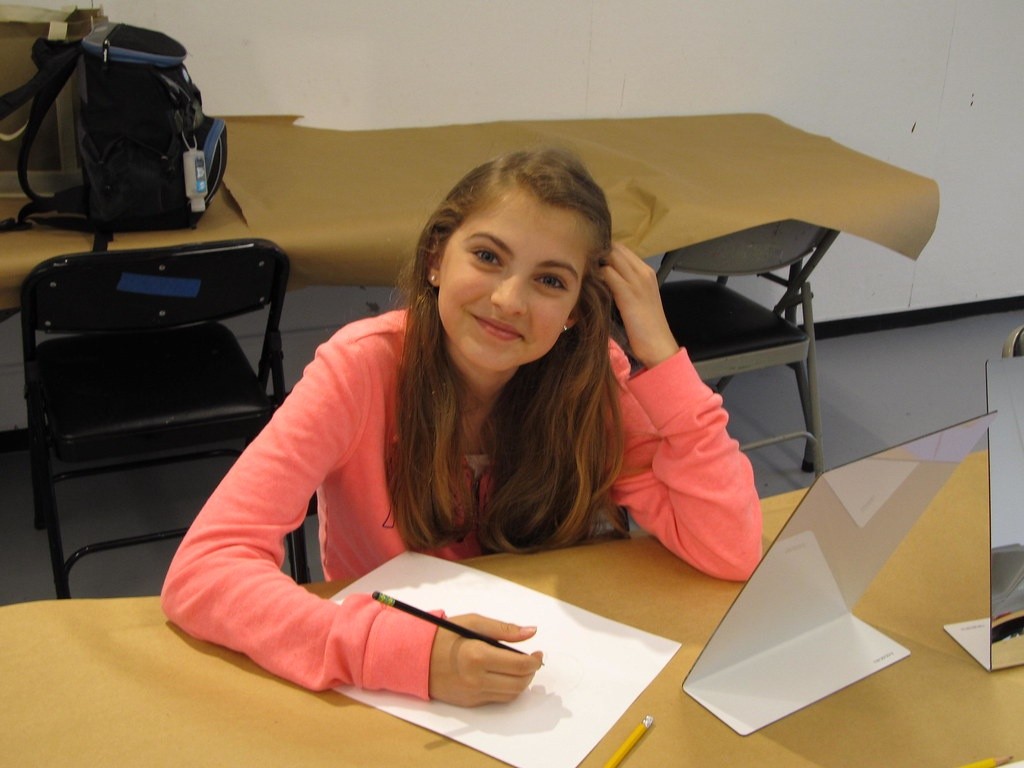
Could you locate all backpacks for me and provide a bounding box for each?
[0,21,228,253]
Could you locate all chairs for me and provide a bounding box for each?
[19,238,312,601]
[610,218,841,538]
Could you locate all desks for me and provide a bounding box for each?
[0,450,1024,768]
[0,113,942,295]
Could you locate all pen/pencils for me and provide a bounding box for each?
[606,715,655,768]
[371,591,548,666]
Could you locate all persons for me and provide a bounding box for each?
[158,149,765,711]
